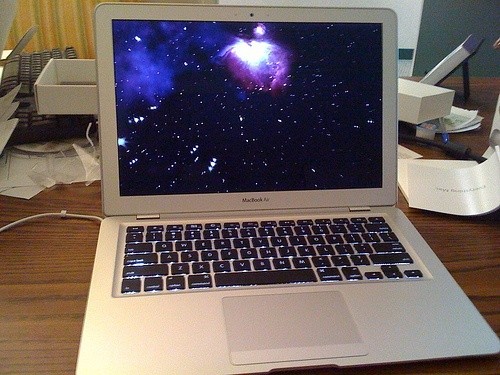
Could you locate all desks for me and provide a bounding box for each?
[0,71,499,375]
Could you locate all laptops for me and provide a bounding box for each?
[75,1,496,374]
